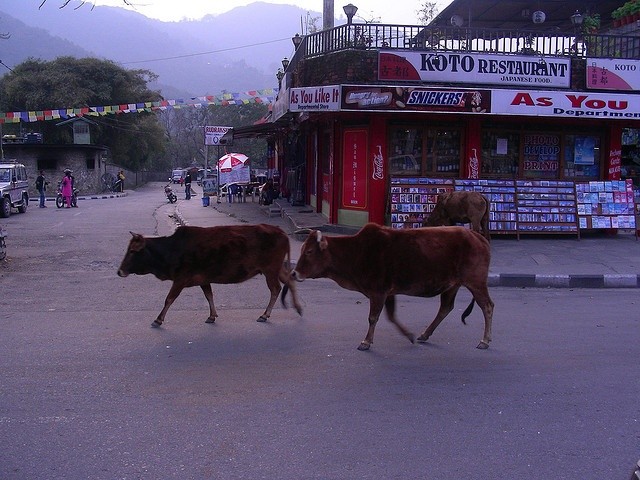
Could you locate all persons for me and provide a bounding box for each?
[117,175,124,192]
[35,169,51,208]
[410,214,416,222]
[392,215,396,222]
[180,176,184,186]
[61,169,73,208]
[58,169,78,207]
[417,214,422,222]
[399,215,403,222]
[392,205,397,212]
[121,171,123,175]
[404,215,408,222]
[262,178,274,204]
[185,174,191,200]
[118,172,125,182]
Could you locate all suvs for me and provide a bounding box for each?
[0,159,28,218]
[172,175,183,184]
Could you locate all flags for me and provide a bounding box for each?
[0,87,279,126]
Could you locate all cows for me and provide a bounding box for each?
[421,191,491,243]
[117,223,304,328]
[291,223,495,350]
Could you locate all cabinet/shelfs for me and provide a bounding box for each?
[455,177,519,242]
[576,179,638,242]
[515,179,580,241]
[386,177,454,233]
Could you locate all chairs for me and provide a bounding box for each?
[220,184,233,203]
[252,183,267,201]
[228,184,240,204]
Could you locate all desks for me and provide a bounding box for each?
[237,183,259,203]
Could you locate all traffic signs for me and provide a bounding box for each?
[205,126,234,145]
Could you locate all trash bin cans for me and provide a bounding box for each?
[202,193,210,207]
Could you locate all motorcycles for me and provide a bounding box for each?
[161,181,177,204]
[56,181,79,208]
